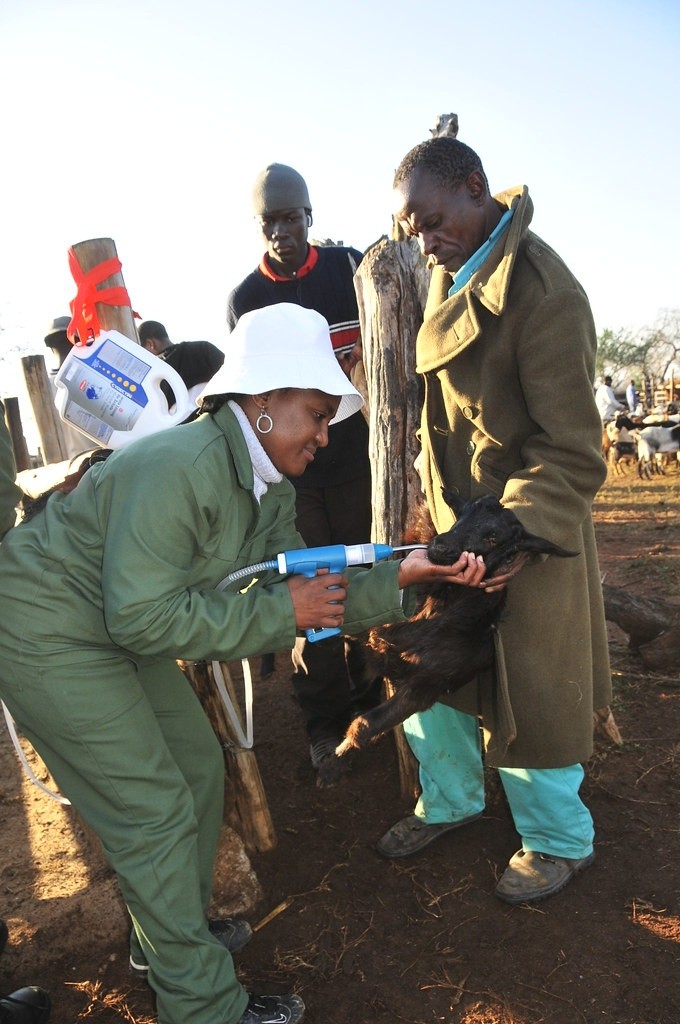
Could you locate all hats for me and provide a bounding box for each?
[44,315,80,345]
[254,162,313,214]
[196,303,365,427]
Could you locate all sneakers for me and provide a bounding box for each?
[309,737,342,768]
[127,918,252,978]
[376,812,484,858]
[495,845,595,903]
[238,991,305,1024]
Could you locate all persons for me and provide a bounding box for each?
[0,300,486,1024]
[134,137,636,903]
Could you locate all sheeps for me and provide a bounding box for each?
[605,400,680,480]
[333,487,581,757]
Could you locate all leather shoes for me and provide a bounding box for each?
[0,986,50,1024]
